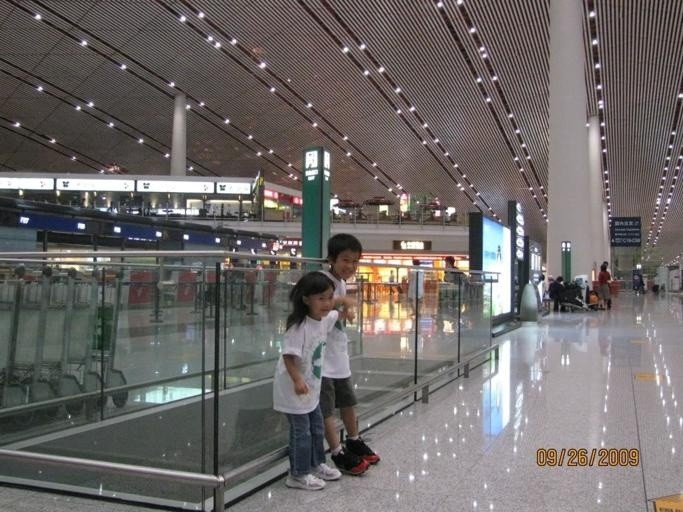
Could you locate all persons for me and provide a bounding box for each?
[442,255,468,328]
[319,232,384,477]
[653,272,660,297]
[406,258,424,318]
[271,270,357,493]
[388,270,395,296]
[602,261,612,310]
[598,264,611,310]
[537,272,590,313]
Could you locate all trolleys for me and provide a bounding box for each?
[0,260,130,429]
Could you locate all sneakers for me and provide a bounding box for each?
[284,469,325,491]
[346,435,380,464]
[312,463,343,481]
[331,446,369,476]
